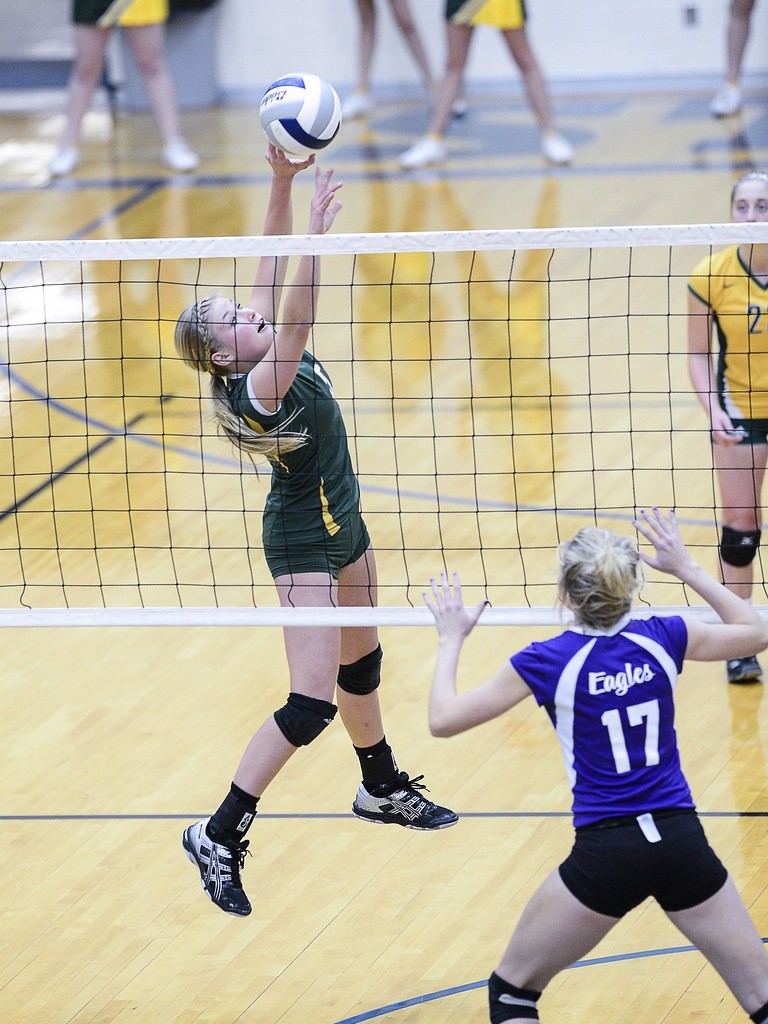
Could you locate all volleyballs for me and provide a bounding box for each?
[255,71,346,160]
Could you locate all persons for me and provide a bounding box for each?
[45,1,759,180]
[421,504,767,1023]
[686,170,768,687]
[173,142,461,922]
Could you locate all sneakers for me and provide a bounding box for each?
[182,816,253,917]
[727,656,762,682]
[351,771,459,831]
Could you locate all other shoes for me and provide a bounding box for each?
[51,147,79,175]
[341,92,372,116]
[541,132,575,164]
[162,140,199,172]
[399,136,444,168]
[449,98,468,116]
[711,82,743,115]
